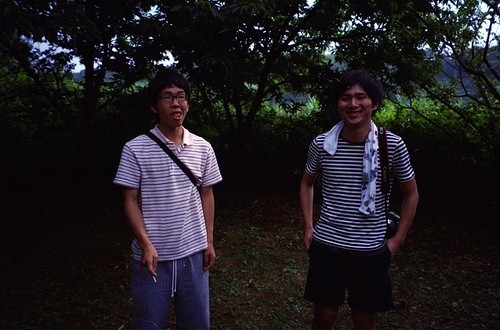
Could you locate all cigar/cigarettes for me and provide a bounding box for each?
[152,275,157,283]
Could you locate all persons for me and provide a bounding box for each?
[300,70,420,330]
[112,67,223,330]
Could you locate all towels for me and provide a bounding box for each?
[323,116,379,216]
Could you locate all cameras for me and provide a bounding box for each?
[387,212,400,238]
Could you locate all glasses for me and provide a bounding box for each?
[155,93,189,102]
